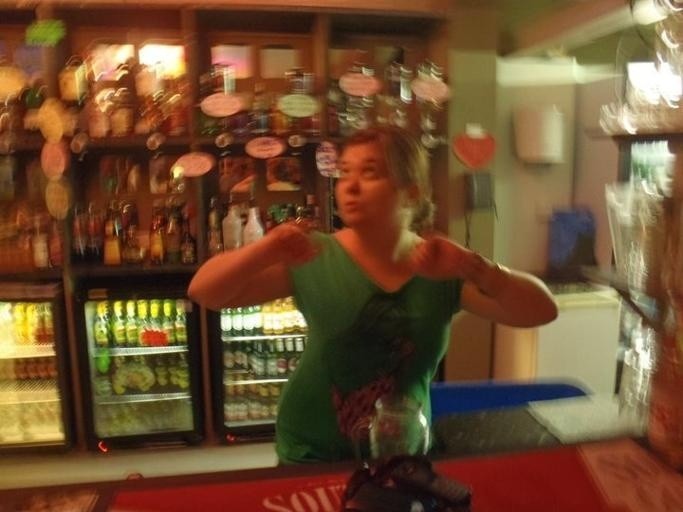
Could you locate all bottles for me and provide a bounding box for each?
[225,301,306,423]
[14,192,322,273]
[92,300,189,395]
[2,301,60,348]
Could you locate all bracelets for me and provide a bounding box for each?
[478,263,512,298]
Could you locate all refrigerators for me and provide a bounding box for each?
[216,293,313,441]
[0,283,70,458]
[74,281,209,454]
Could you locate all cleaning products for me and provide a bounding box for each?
[243,209,264,245]
[222,203,242,251]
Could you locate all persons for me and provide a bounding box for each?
[187,124,558,466]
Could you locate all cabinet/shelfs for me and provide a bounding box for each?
[0,1,450,281]
